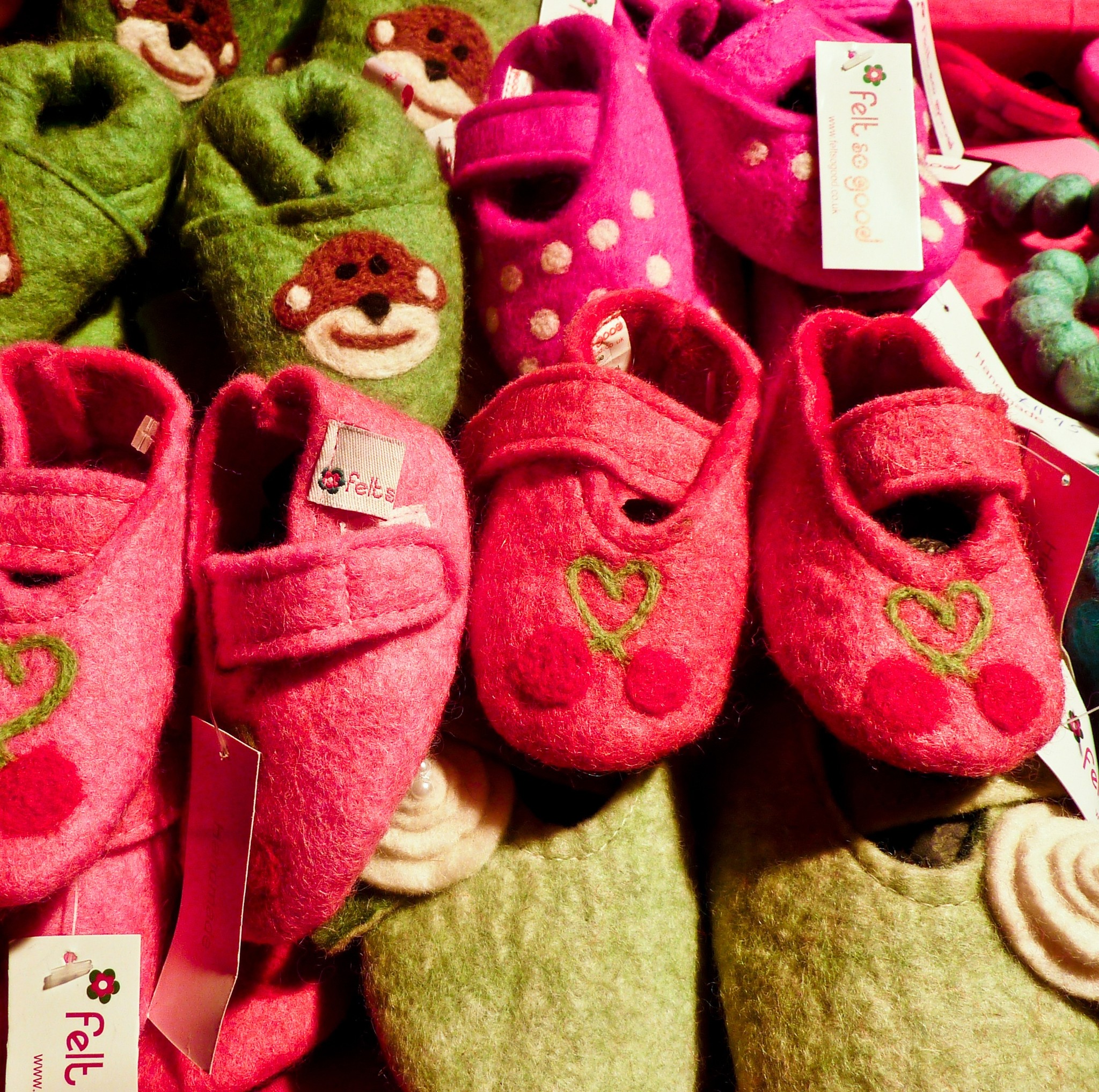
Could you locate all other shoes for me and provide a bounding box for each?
[191,363,470,945]
[753,309,1064,780]
[0,336,188,908]
[181,58,464,433]
[1,36,184,344]
[708,672,1096,1090]
[457,289,763,777]
[648,3,966,294]
[9,837,319,1091]
[312,1,542,136]
[58,0,304,110]
[296,757,705,1090]
[449,13,694,382]
